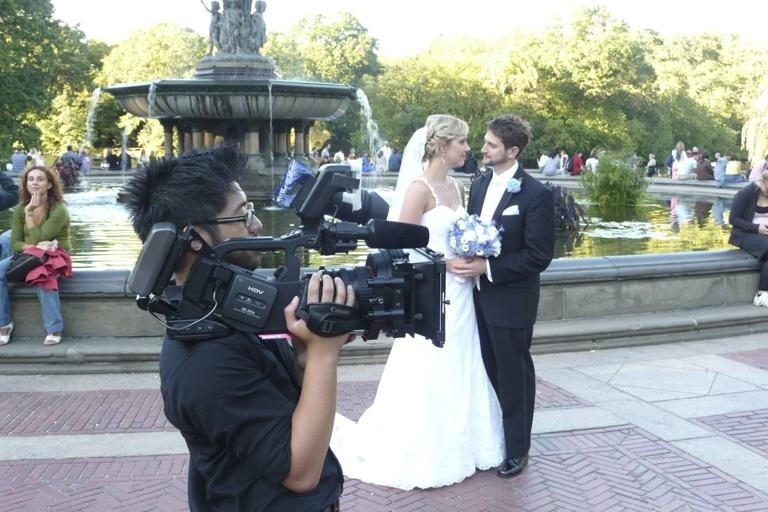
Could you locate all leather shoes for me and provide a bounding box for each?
[498,454,527,477]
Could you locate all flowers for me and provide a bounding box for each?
[505,176,523,193]
[446,213,504,259]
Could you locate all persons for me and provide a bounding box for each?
[668,197,727,232]
[729,173,767,305]
[455,151,485,173]
[539,139,767,182]
[201,0,269,58]
[283,141,401,171]
[125,140,356,512]
[381,112,508,491]
[13,145,157,173]
[0,161,74,349]
[469,112,557,481]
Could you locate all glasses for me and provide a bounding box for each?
[191,202,256,227]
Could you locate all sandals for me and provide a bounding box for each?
[44,333,61,345]
[1,321,15,345]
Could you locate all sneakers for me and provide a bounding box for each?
[753,291,768,307]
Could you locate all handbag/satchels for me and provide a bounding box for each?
[566,157,576,170]
[664,154,673,168]
[6,251,47,281]
